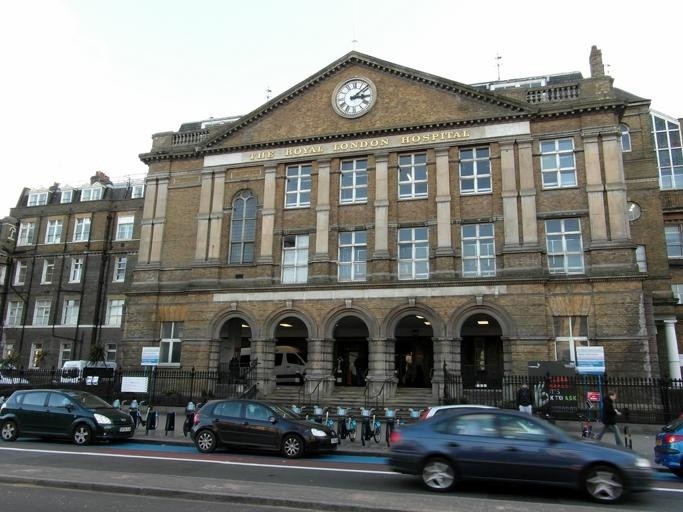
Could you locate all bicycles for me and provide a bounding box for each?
[112,399,159,430]
[291,404,427,448]
[579,415,598,439]
[183,402,196,437]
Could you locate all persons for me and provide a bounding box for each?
[595,390,624,447]
[335,355,345,369]
[516,381,534,416]
[229,354,239,376]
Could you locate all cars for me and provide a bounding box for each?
[382,409,651,505]
[190,399,341,459]
[0,388,135,446]
[419,404,546,442]
[0,363,29,384]
[654,415,683,477]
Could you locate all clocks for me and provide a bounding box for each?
[331,76,378,119]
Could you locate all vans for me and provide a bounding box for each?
[239,345,307,386]
[60,360,117,386]
